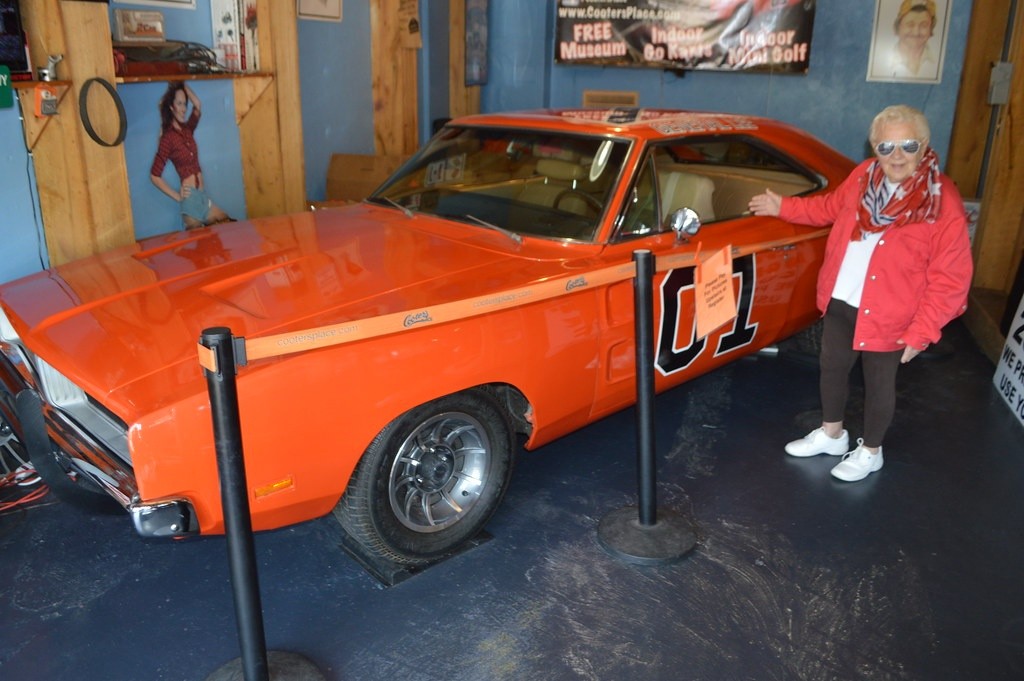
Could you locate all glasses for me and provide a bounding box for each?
[875,139,921,158]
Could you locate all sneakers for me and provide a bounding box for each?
[830,438,884,481]
[784,427,850,457]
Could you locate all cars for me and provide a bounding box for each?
[0,109,878,563]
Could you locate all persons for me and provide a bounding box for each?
[873,0,938,80]
[148,80,231,232]
[748,104,974,481]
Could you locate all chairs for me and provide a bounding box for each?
[646,173,717,224]
[516,158,586,215]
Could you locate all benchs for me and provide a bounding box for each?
[635,165,816,219]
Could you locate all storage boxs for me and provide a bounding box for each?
[326,153,420,208]
[113,9,165,42]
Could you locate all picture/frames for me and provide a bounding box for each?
[866,0,952,84]
